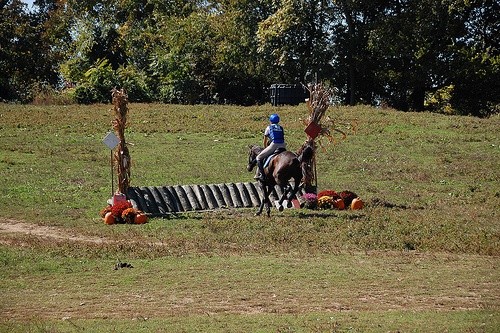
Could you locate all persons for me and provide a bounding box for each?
[253,114,287,178]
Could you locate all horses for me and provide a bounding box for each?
[246,144,314,218]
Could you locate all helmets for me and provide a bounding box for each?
[269,114,281,123]
